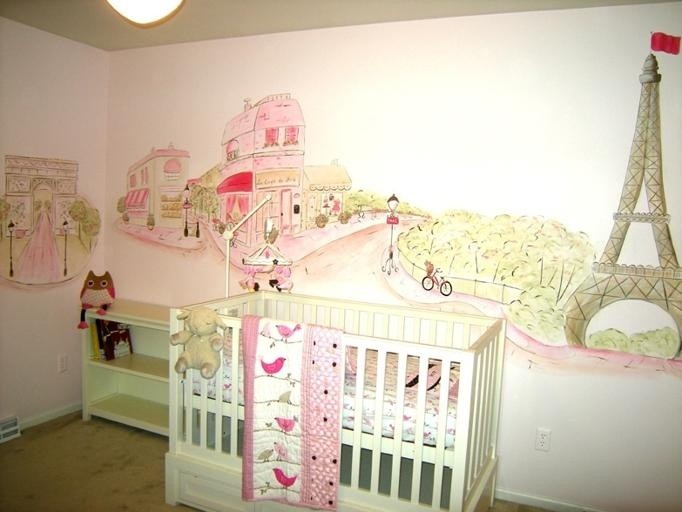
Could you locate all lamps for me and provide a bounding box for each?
[105,0,186,27]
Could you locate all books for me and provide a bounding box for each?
[86,318,134,361]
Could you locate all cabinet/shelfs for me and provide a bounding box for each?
[81,297,168,438]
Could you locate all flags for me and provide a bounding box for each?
[648,30,682,56]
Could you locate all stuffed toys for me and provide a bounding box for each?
[75,268,116,329]
[169,309,227,379]
[238,240,293,295]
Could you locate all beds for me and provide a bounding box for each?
[164,290,506,512]
